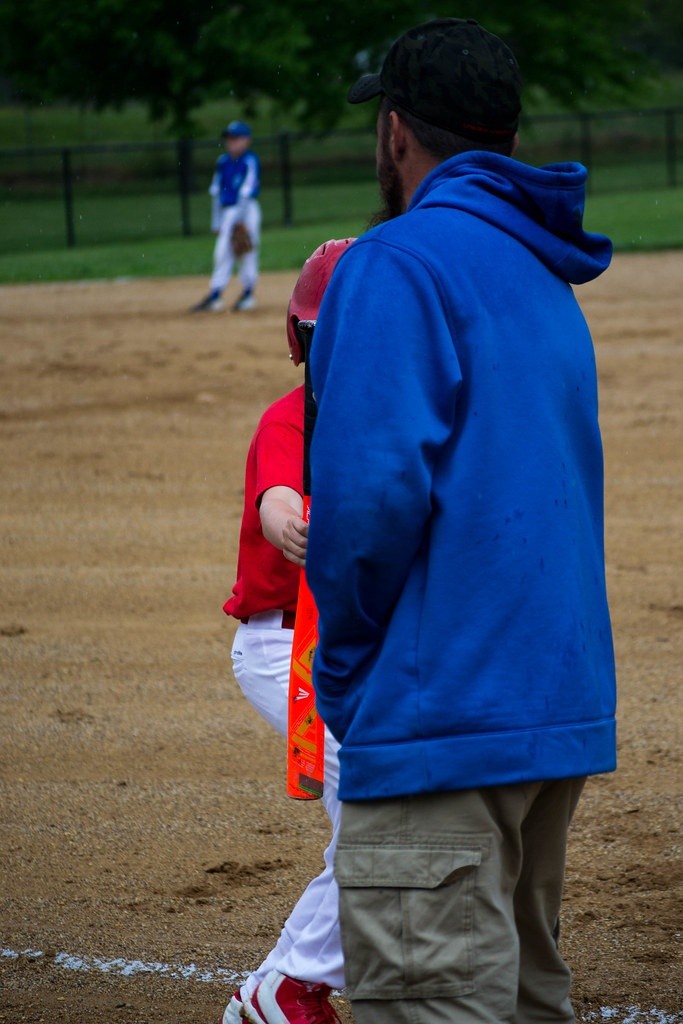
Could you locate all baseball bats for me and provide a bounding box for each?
[286,313,332,802]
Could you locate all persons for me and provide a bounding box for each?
[306,18,616,1024]
[192,119,264,314]
[222,236,364,1024]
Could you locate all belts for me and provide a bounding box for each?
[241,611,295,629]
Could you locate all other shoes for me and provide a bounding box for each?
[234,296,256,311]
[193,296,224,313]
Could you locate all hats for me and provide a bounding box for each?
[219,121,250,139]
[348,17,522,140]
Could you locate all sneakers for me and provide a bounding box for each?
[239,970,342,1024]
[222,990,250,1024]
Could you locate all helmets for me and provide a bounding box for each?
[286,237,360,367]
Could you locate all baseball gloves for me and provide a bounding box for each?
[232,221,251,256]
